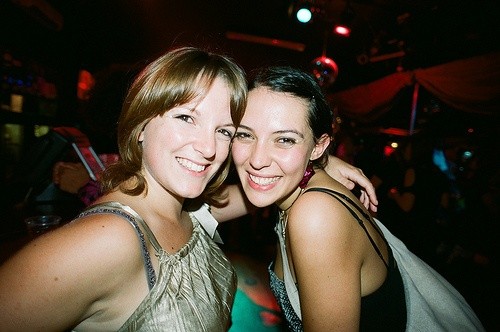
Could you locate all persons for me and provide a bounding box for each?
[0,47,379,331]
[231,68,408,332]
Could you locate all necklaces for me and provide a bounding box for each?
[281,188,302,216]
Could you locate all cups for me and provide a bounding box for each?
[24,215,62,239]
